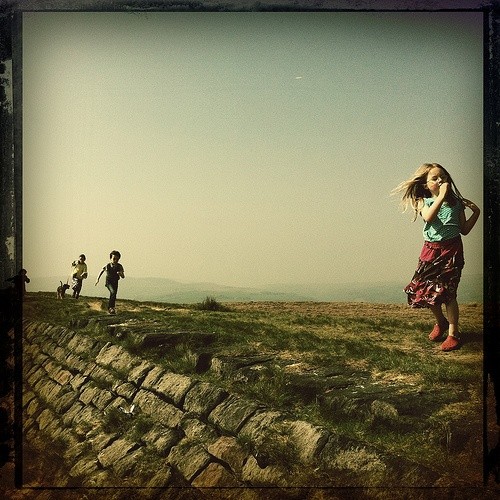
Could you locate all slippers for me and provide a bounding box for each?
[441,335,459,351]
[428,321,449,342]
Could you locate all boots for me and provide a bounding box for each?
[76,294,79,299]
[72,292,76,298]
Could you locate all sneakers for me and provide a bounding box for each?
[108,307,115,314]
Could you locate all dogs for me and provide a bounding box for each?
[57,280,70,299]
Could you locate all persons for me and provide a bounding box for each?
[71,254,88,300]
[4,269,30,301]
[95,250,125,314]
[388,163,480,350]
